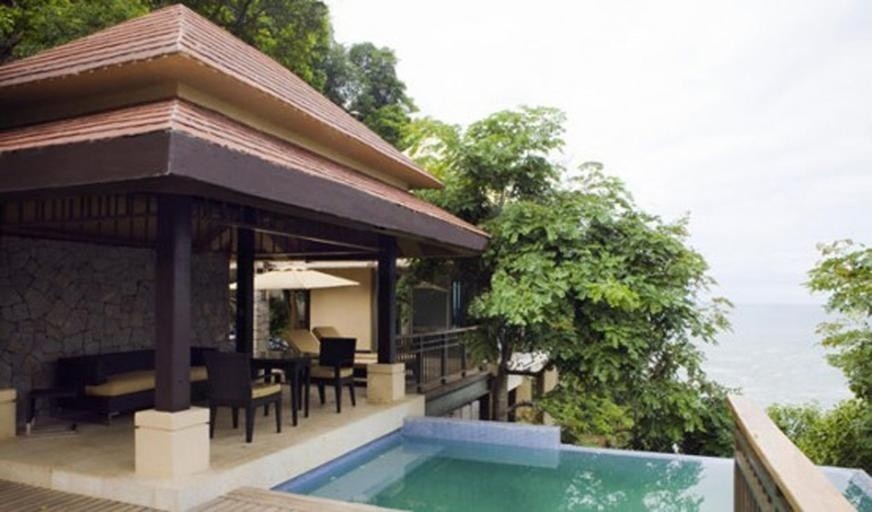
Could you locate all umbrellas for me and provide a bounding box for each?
[224,268,361,330]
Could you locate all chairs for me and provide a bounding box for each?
[205,326,377,444]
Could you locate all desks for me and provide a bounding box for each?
[25,388,79,434]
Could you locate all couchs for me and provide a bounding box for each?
[55,347,218,425]
